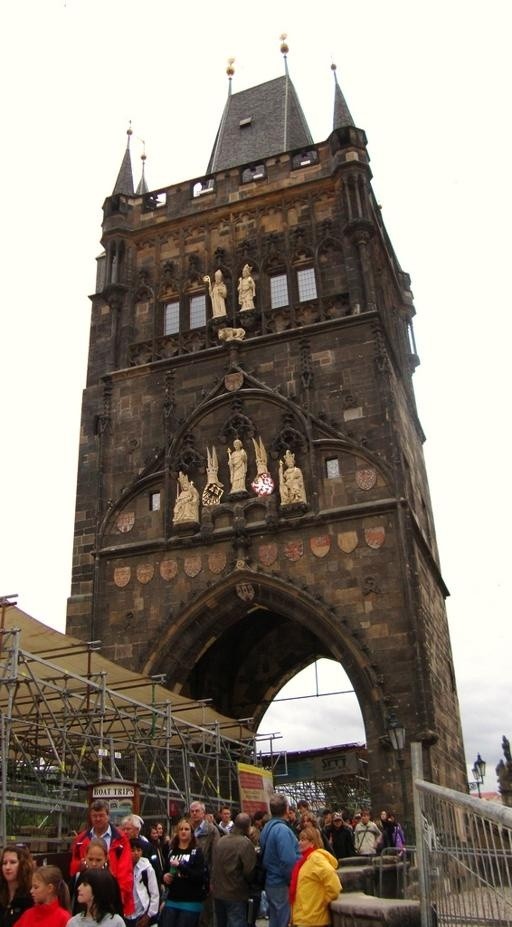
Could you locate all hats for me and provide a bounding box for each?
[333,813,343,821]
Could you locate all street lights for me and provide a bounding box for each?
[469,750,486,798]
[384,707,413,847]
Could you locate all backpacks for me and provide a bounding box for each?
[195,863,211,901]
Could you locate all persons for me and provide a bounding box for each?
[238,264,257,311]
[2,794,408,927]
[227,440,248,494]
[280,451,306,504]
[173,471,199,524]
[206,270,228,317]
